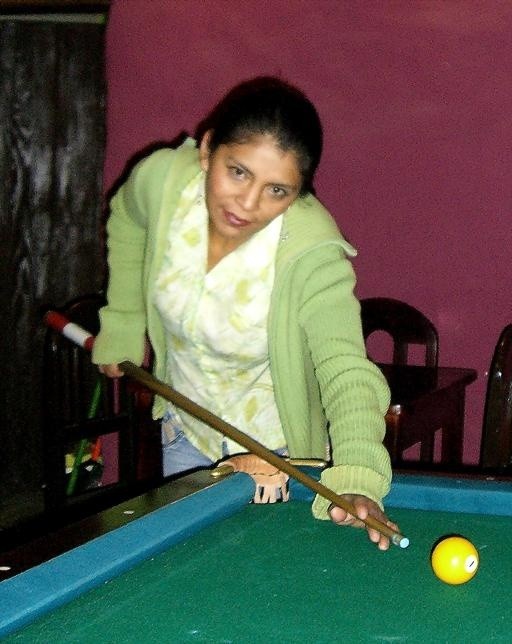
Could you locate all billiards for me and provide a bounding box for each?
[430,536,480,584]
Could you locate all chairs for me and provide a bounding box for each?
[429,324,511,480]
[358,298,437,470]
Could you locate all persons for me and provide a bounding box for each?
[89,76,399,551]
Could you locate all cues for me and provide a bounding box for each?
[45,311,409,548]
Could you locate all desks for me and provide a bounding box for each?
[0,453,512,644]
[371,363,478,470]
